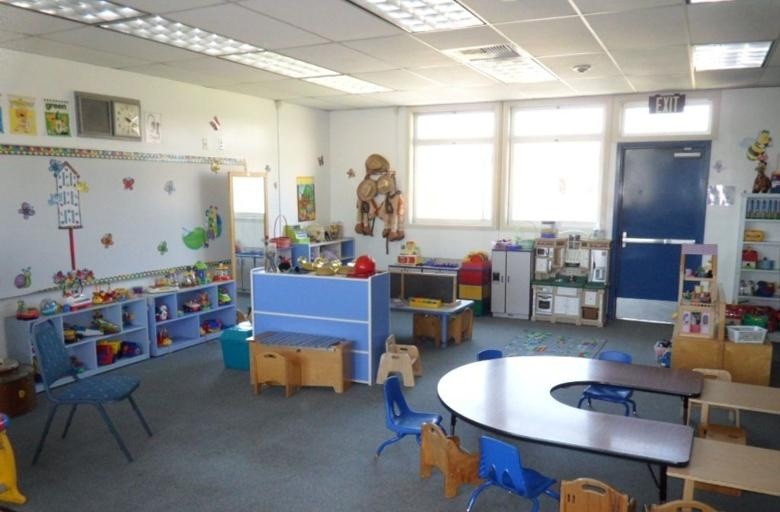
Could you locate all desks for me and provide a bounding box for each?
[437,355,704,505]
[686,378,780,425]
[667,437,780,512]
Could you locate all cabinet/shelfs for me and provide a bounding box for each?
[5,236,614,396]
[671,193,780,407]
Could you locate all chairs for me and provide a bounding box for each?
[466,435,560,512]
[576,350,637,417]
[31,319,153,466]
[686,368,741,429]
[560,477,637,512]
[420,422,488,498]
[376,376,448,455]
[642,500,716,512]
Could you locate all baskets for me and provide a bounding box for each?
[269,214,291,248]
[726,325,767,343]
[314,264,337,275]
[338,265,355,274]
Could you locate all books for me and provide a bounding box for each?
[305,219,341,263]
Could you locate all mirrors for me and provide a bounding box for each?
[226,169,272,324]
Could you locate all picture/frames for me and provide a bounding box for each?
[74,91,113,139]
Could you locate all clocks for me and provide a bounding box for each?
[113,96,144,142]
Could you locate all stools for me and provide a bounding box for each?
[694,424,747,497]
[0,365,38,418]
